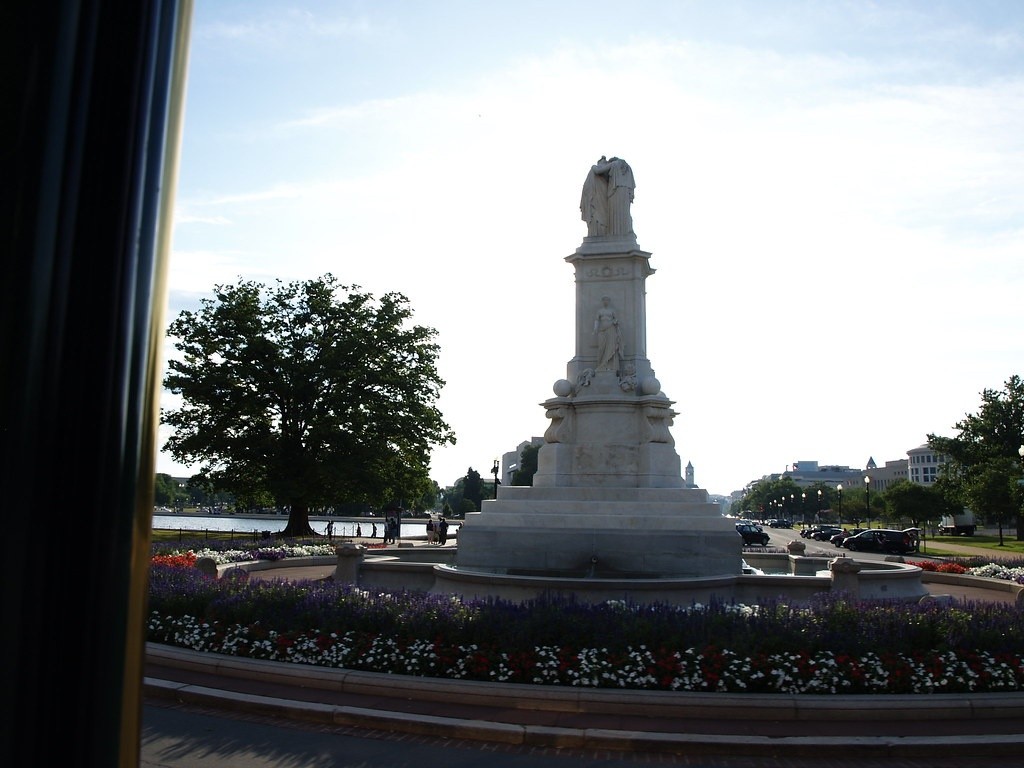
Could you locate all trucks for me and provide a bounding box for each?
[937,504,978,537]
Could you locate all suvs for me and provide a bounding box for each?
[842,527,924,555]
[830,528,867,547]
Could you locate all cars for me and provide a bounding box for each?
[736,524,770,547]
[735,518,792,530]
[800,524,844,541]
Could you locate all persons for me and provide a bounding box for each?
[878,523,906,531]
[592,294,623,373]
[456,522,463,544]
[371,522,377,537]
[383,518,397,545]
[325,520,334,537]
[840,529,848,546]
[920,528,924,539]
[580,155,636,237]
[426,515,449,546]
[356,523,361,537]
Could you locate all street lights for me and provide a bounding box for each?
[790,495,795,519]
[837,484,843,525]
[817,489,821,524]
[781,496,785,519]
[801,493,806,524]
[864,475,871,528]
